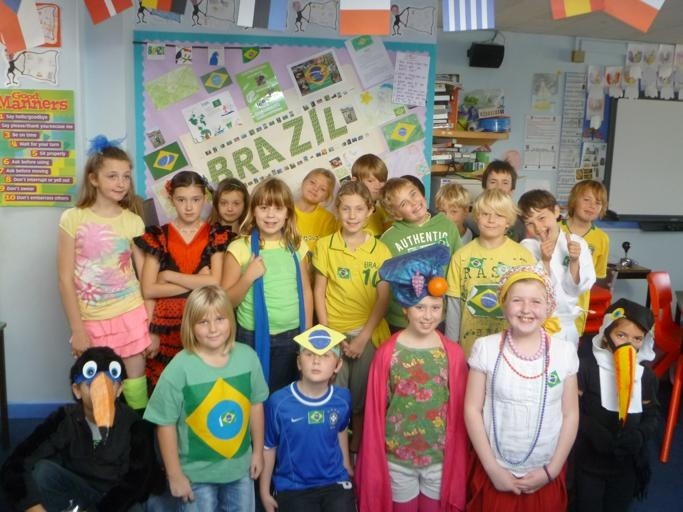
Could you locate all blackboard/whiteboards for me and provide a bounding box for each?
[602,92,683,221]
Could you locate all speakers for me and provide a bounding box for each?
[469,41,504,68]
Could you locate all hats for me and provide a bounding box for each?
[602,298,655,334]
[379,244,450,307]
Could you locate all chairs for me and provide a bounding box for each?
[646,271,683,464]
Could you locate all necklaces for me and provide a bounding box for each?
[489,325,551,466]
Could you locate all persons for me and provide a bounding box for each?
[4,347,160,512]
[566,298,663,510]
[57,146,160,457]
[463,267,581,512]
[207,155,621,451]
[258,325,356,511]
[141,285,270,512]
[132,172,236,401]
[354,245,472,512]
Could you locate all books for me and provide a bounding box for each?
[434,74,463,130]
[431,138,477,165]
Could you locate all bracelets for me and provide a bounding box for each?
[543,465,554,484]
